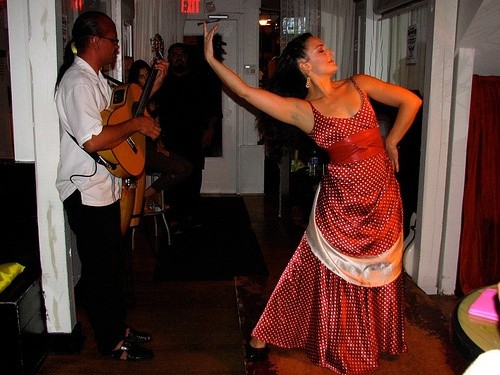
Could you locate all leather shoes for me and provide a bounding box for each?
[246,342,268,359]
[123,327,153,344]
[104,340,153,362]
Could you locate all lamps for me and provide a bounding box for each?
[197,15,229,25]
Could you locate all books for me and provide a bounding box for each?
[468,288,500,321]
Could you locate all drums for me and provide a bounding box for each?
[120,173,136,235]
[129,171,146,227]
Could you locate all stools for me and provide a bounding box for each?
[126,155,170,250]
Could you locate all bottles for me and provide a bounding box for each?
[310,148,319,171]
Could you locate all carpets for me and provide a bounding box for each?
[153,196,269,280]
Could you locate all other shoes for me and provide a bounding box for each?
[182,214,204,228]
[166,219,184,235]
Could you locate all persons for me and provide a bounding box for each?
[202,20,422,375]
[119,59,184,235]
[54,10,169,362]
[123,55,134,84]
[152,41,217,230]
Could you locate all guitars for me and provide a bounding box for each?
[95,32,165,179]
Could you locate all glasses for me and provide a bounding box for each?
[87,34,119,45]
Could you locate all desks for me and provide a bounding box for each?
[451,282,500,362]
